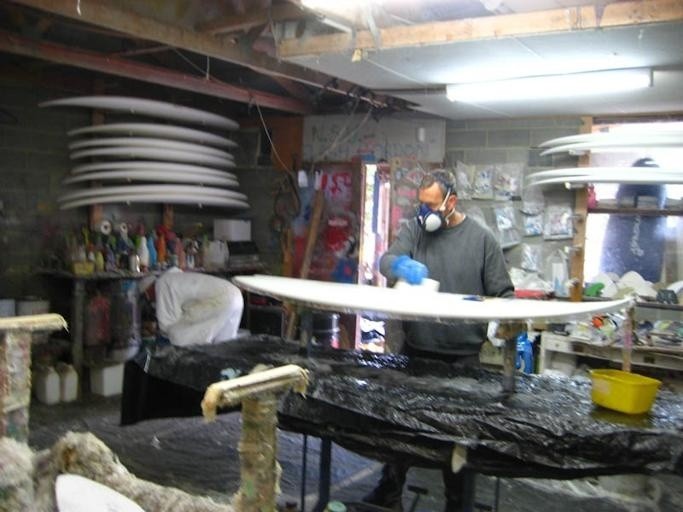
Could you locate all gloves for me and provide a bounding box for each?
[516,332,534,373]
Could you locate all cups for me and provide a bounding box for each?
[568,285,583,301]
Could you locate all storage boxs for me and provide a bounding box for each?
[586,366,662,415]
[90,362,126,396]
[214,218,252,242]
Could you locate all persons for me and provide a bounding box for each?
[361,168,523,510]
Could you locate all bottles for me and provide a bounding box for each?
[129,219,197,272]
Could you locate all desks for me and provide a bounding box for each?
[42,264,266,392]
[118,332,682,511]
[536,331,683,381]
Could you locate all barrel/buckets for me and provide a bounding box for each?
[35,365,60,405]
[56,362,77,404]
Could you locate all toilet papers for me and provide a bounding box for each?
[91,220,111,235]
[112,224,128,232]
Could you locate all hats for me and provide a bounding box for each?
[138,275,156,294]
